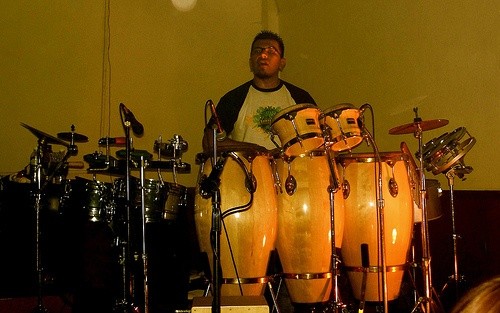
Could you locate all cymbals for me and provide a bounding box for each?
[17,120,71,147]
[389,118,450,136]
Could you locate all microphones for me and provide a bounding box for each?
[211,101,227,141]
[124,105,144,136]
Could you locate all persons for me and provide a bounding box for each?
[203,30,317,154]
[450,276,500,313]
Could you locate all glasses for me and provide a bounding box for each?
[251,45,282,58]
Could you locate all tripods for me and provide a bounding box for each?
[316,145,351,313]
[407,122,471,313]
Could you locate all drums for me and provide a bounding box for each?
[137,178,190,223]
[414,126,476,176]
[270,147,346,303]
[337,150,415,302]
[270,103,324,158]
[319,103,365,153]
[59,178,113,219]
[192,144,278,299]
[422,178,443,222]
[114,175,151,210]
